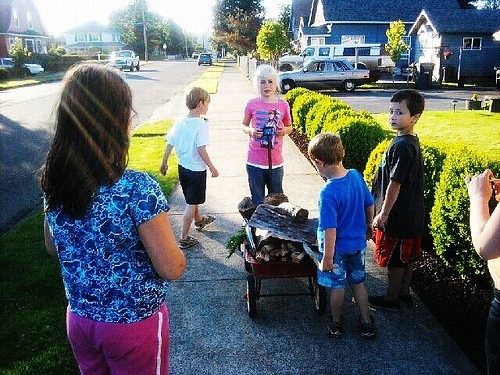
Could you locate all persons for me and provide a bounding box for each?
[239,63,294,214]
[464,169,500,375]
[160,85,219,250]
[370,88,424,315]
[36,61,186,375]
[306,131,378,341]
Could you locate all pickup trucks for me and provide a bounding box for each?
[278,43,383,72]
[109,50,140,71]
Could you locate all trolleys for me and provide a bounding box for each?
[244,201,328,317]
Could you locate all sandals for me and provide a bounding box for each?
[178,236,199,249]
[194,214,216,231]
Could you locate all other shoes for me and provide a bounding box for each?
[359,314,376,338]
[326,314,344,338]
[368,295,400,312]
[398,294,414,306]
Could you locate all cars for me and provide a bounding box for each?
[192,52,199,59]
[0,58,44,76]
[279,60,370,93]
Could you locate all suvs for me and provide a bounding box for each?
[198,53,212,66]
